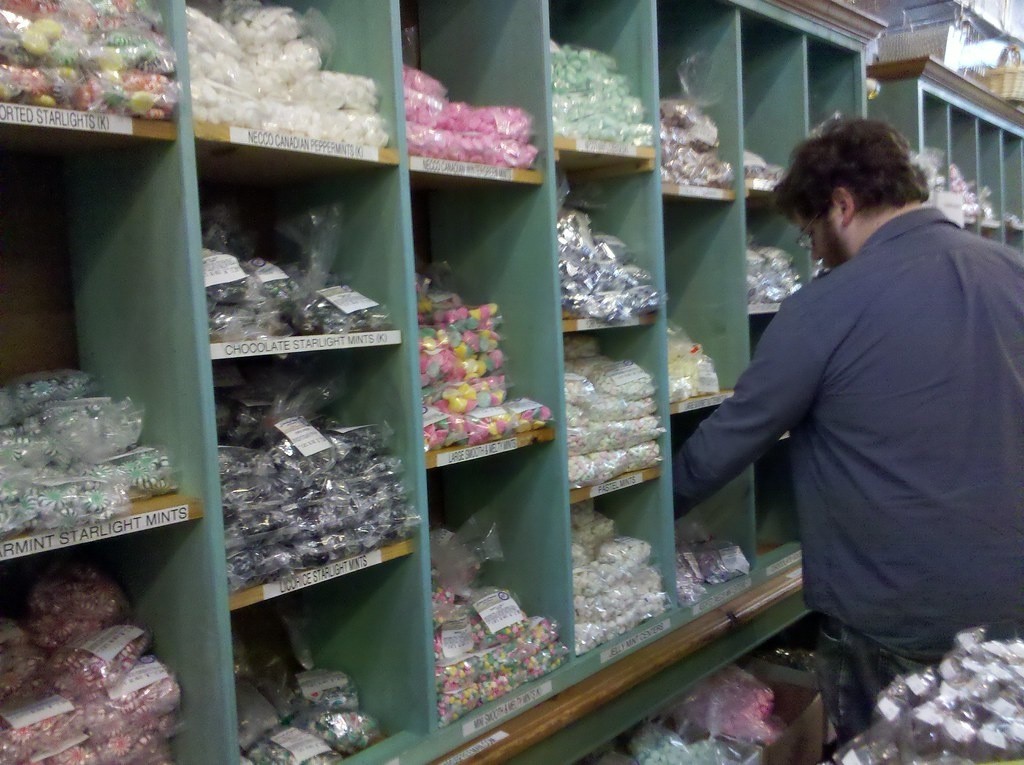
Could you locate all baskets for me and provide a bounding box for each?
[986,44,1024,101]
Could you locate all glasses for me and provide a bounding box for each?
[795,189,838,250]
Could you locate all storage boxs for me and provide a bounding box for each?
[659,714,763,765]
[758,678,825,765]
[759,661,837,745]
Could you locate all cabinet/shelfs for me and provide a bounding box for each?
[0,0,1024,765]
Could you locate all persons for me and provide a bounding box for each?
[672,117,1024,742]
